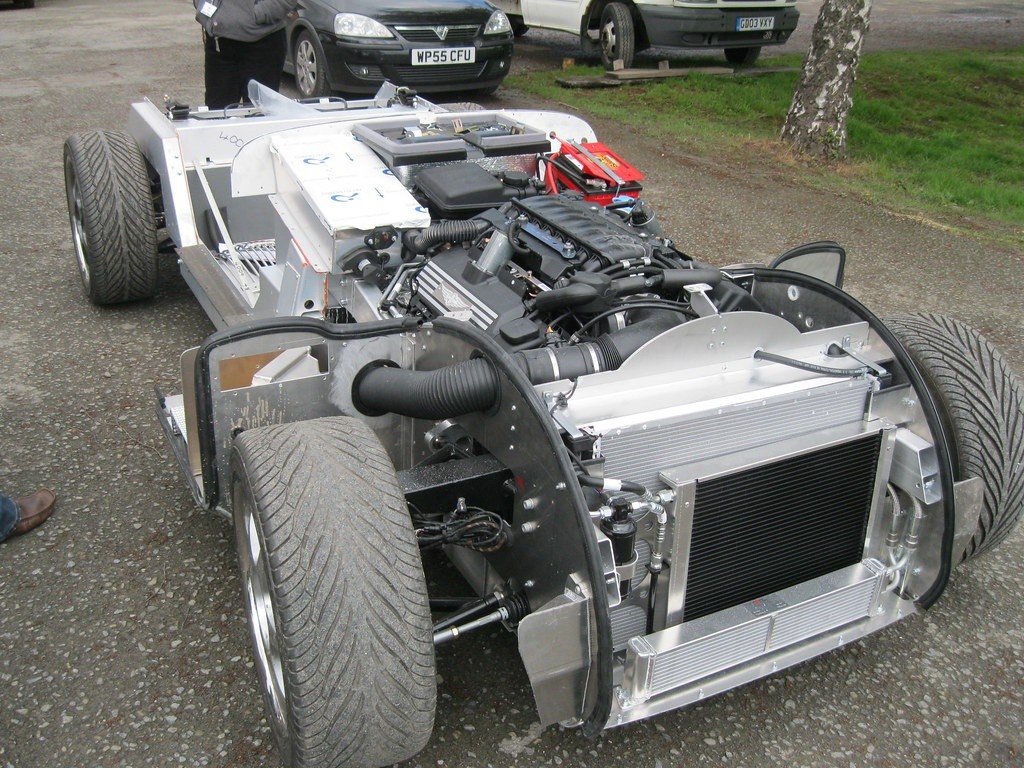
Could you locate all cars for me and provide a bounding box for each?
[201,0,517,100]
[487,0,804,71]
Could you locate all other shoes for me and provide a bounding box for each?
[10,488,56,537]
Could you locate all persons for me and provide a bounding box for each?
[192,0,296,109]
[0,487,56,544]
